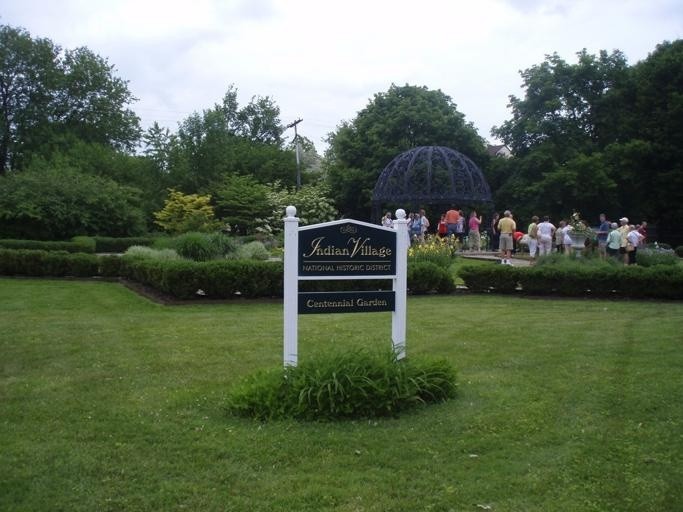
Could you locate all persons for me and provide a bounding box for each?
[381,204,648,266]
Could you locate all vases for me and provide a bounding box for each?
[567,231,587,250]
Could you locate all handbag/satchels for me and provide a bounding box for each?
[626,243,634,252]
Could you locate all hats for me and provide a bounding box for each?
[619,217,628,223]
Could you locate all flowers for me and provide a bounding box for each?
[568,207,592,242]
